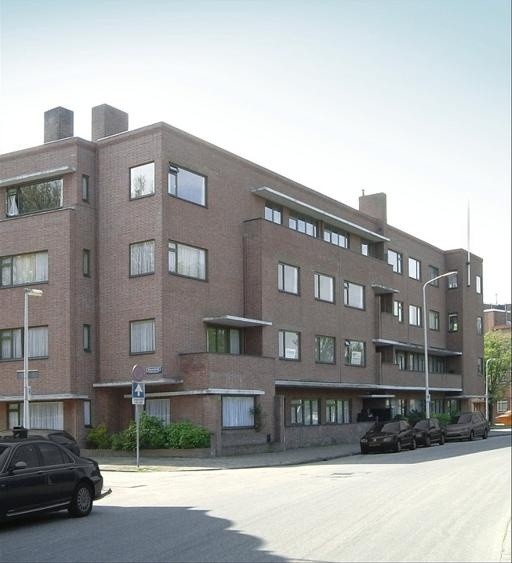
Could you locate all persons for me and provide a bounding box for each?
[356,407,369,422]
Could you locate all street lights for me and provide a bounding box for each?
[422,271,458,418]
[23,287,43,429]
[485,358,495,422]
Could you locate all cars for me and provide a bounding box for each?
[360,410,490,454]
[0,424,103,522]
